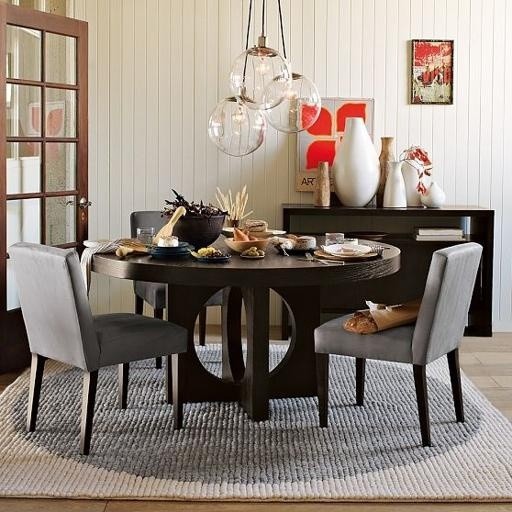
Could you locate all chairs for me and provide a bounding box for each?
[6,239,190,456]
[129,208,242,371]
[310,241,482,447]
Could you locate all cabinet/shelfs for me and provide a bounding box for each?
[283,204,497,339]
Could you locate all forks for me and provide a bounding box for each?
[305,250,329,267]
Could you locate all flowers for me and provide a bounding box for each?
[399,141,434,195]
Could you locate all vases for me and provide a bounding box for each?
[382,160,407,208]
[313,161,332,207]
[328,118,383,208]
[420,182,445,208]
[376,136,396,205]
[401,161,423,208]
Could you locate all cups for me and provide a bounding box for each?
[137,227,155,242]
[326,232,345,245]
[159,236,179,247]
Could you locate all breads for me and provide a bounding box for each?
[245,220,267,232]
[344,312,378,334]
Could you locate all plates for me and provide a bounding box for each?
[275,245,317,255]
[357,232,391,242]
[323,244,372,256]
[192,253,230,259]
[314,248,379,260]
[241,254,265,259]
[150,242,194,256]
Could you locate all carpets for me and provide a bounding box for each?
[1,338,512,503]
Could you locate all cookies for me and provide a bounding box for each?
[242,247,264,256]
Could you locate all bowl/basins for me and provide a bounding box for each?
[225,238,271,254]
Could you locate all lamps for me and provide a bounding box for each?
[207,2,322,158]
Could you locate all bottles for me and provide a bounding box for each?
[314,112,446,210]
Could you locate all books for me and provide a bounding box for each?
[410,225,467,242]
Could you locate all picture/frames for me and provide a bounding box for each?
[409,37,456,107]
